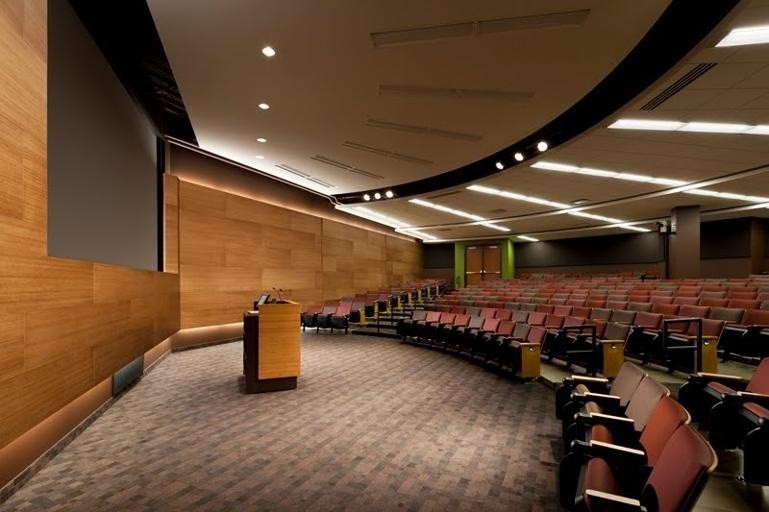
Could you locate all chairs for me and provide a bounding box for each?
[302,278,447,335]
[554,355,769,511]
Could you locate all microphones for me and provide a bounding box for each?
[272,287,282,301]
[279,288,289,303]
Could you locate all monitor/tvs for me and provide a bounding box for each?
[256,293,270,308]
